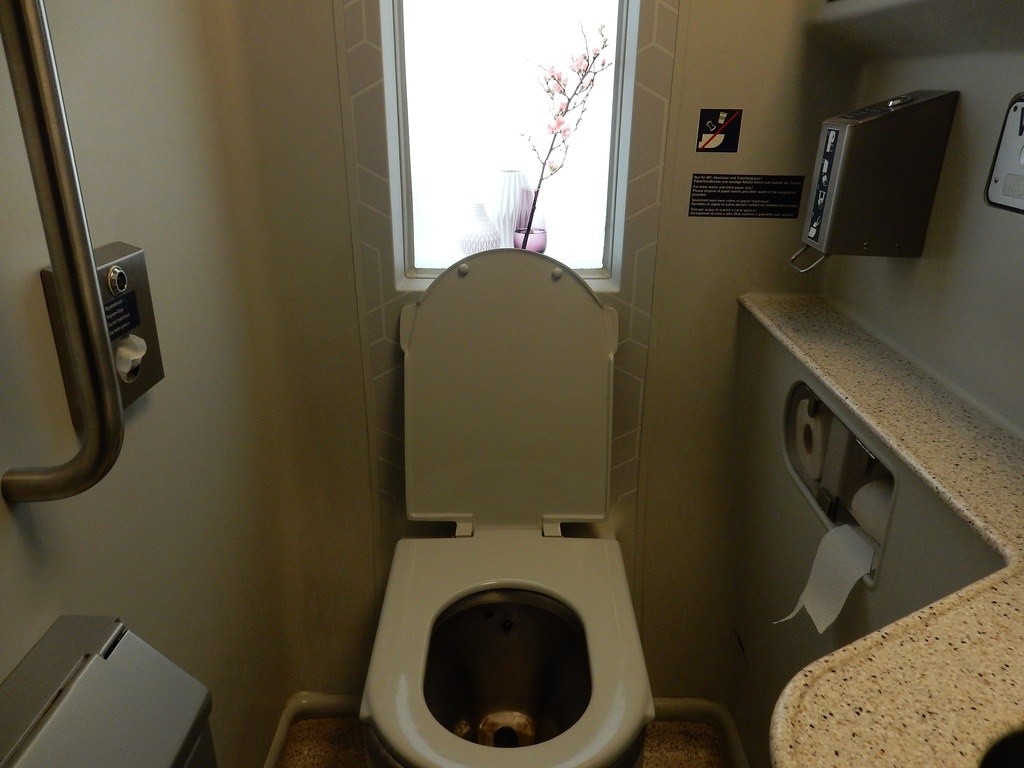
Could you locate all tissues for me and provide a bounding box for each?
[40,241,167,427]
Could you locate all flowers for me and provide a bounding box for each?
[509,16,612,228]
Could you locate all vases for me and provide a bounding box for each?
[513,227,547,253]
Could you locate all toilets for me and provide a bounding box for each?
[358,247,656,767]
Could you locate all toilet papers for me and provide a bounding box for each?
[791,398,830,479]
[774,476,895,637]
